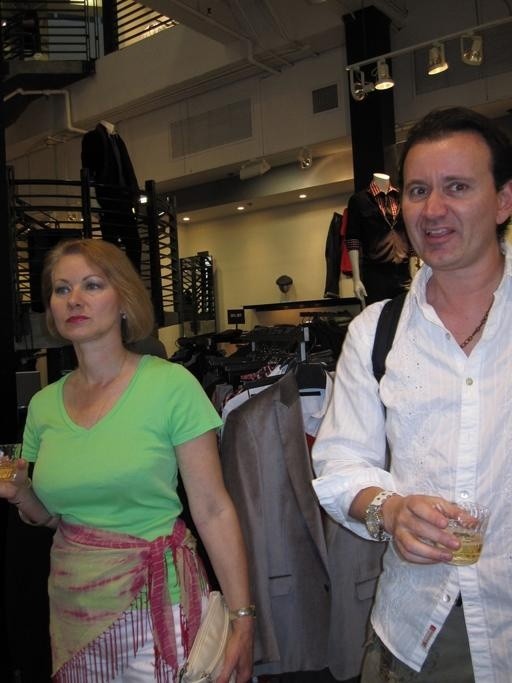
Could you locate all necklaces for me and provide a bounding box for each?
[459,294,495,350]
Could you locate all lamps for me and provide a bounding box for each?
[296,145,313,170]
[236,158,271,180]
[346,27,485,104]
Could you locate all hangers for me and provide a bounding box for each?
[166,324,327,390]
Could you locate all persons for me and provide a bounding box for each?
[77,117,143,273]
[1,237,257,682]
[305,105,512,683]
[345,169,413,309]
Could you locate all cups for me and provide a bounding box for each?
[434,500,491,568]
[1,443,20,484]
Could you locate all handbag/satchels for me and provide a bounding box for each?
[174,589,239,683]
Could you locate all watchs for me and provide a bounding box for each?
[364,486,398,546]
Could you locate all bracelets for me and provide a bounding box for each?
[8,476,32,506]
[16,506,54,527]
[226,602,257,622]
